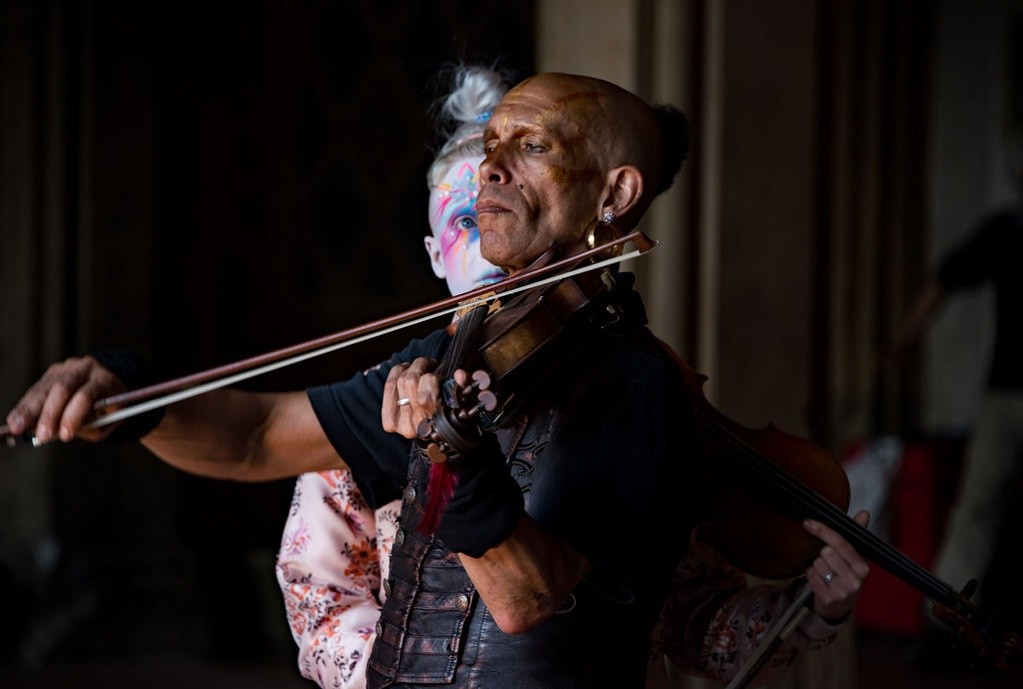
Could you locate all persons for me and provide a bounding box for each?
[833,201,1023,689]
[273,59,868,689]
[6,70,694,689]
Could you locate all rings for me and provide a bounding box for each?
[396,398,409,406]
[821,570,836,584]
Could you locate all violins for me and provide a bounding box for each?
[417,256,607,463]
[655,334,1023,661]
